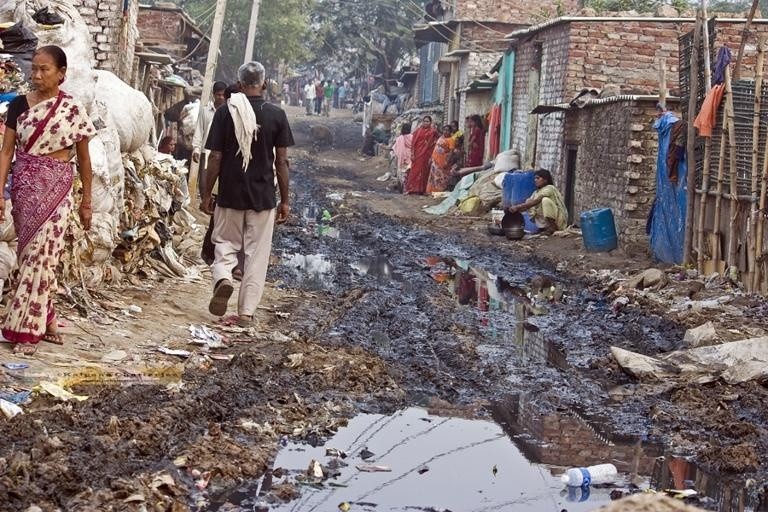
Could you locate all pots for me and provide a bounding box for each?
[501,207,526,230]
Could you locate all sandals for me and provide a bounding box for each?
[43,331,64,345]
[13,342,38,354]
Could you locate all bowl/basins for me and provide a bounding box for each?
[504,227,525,240]
[488,221,504,235]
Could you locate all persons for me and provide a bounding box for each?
[391,115,486,197]
[0,45,98,355]
[508,169,568,235]
[159,61,295,327]
[303,76,350,117]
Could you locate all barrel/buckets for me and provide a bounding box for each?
[579,208,617,253]
[503,169,537,231]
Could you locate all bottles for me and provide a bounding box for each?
[561,463,618,487]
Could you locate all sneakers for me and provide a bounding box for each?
[209,279,233,316]
[237,316,257,327]
